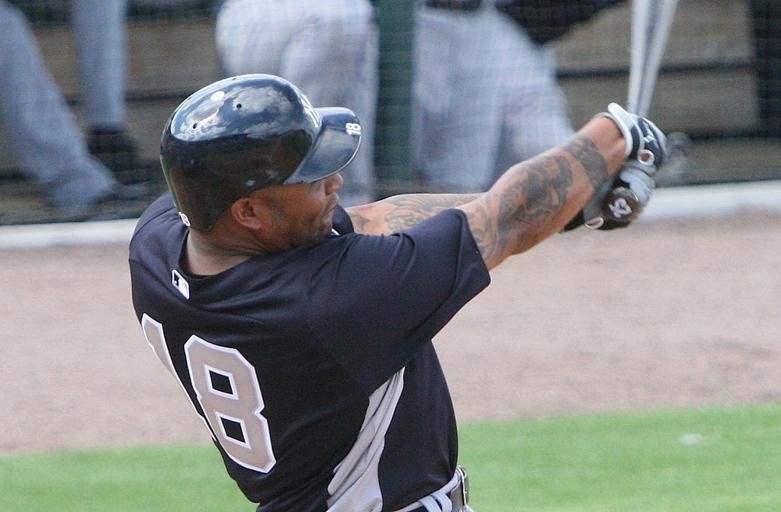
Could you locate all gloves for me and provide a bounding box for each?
[557,102,669,234]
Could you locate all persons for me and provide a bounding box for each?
[125,66,673,510]
[0,1,165,220]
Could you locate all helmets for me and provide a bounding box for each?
[159,71,363,231]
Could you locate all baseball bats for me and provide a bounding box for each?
[600,0,679,223]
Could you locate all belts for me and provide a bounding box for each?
[409,464,469,512]
